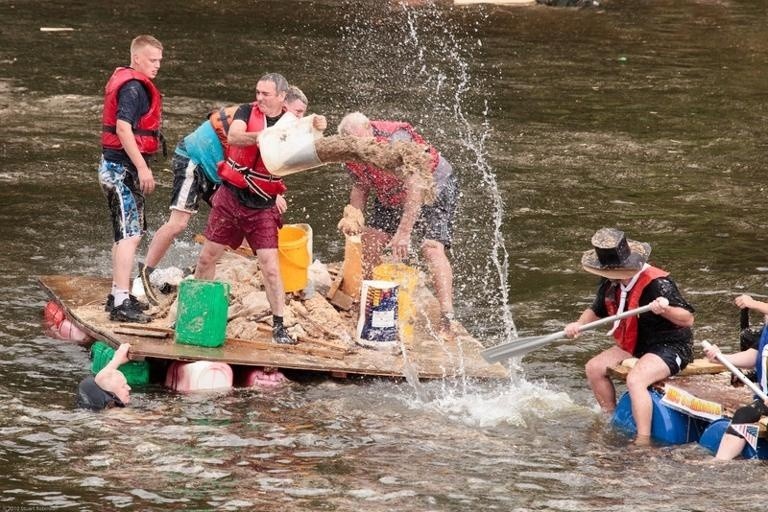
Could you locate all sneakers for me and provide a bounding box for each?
[271,326,297,344]
[439,312,457,341]
[104,294,150,324]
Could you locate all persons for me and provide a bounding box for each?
[337,112,460,346]
[99,34,163,323]
[703,294,768,461]
[75,342,132,409]
[564,227,696,448]
[196,73,328,345]
[131,86,309,296]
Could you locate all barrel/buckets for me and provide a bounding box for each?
[372,263,425,340]
[89,342,150,387]
[43,300,66,327]
[246,367,287,390]
[276,225,312,295]
[257,114,326,180]
[172,276,231,348]
[611,384,712,444]
[52,317,95,346]
[696,417,768,463]
[164,359,234,393]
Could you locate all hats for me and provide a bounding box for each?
[581,227,651,280]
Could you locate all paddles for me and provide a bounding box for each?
[480,297,669,365]
[113,322,344,360]
